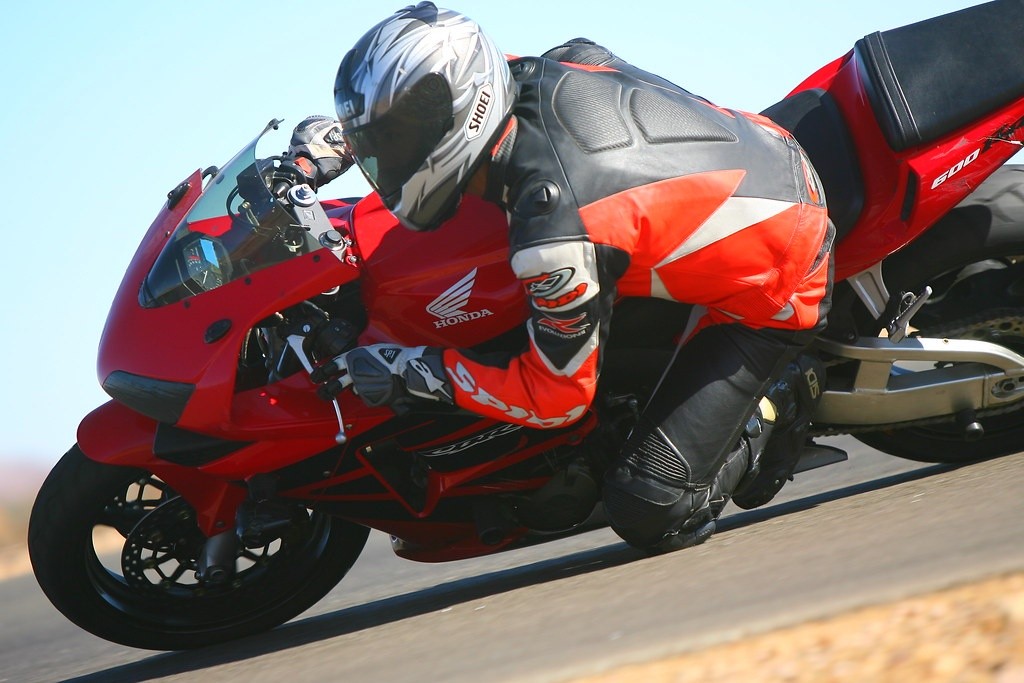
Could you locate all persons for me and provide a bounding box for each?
[289,1,836,552]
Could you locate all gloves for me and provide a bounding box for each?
[288,115,355,187]
[310,343,465,418]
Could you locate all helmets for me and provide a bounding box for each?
[334,1,518,232]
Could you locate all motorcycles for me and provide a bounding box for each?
[26,0,1023,649]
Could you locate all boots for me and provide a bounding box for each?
[731,352,827,509]
